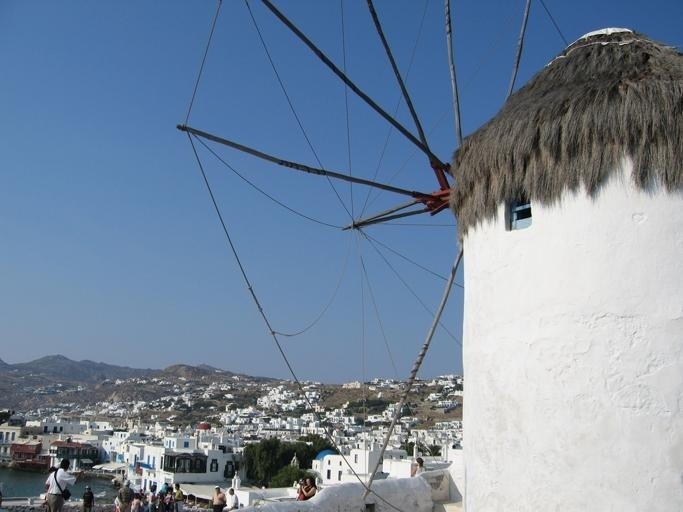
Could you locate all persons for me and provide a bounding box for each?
[43,457,82,511]
[113,479,185,511]
[211,485,225,511]
[295,476,316,500]
[223,488,237,511]
[82,485,94,512]
